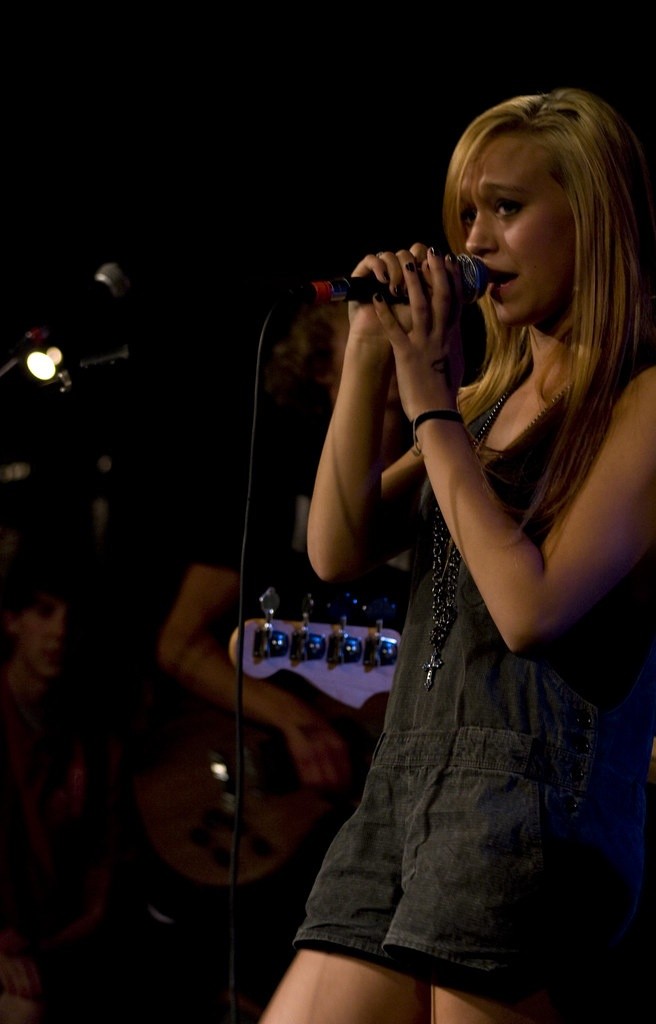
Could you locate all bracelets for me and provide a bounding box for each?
[410,410,464,433]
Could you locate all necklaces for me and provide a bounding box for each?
[421,380,575,692]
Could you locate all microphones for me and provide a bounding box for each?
[286,252,488,306]
[23,261,131,346]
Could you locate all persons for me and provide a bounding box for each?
[0,301,451,1024]
[261,88,656,1024]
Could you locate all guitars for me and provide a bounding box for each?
[228,586,402,721]
[133,707,339,890]
